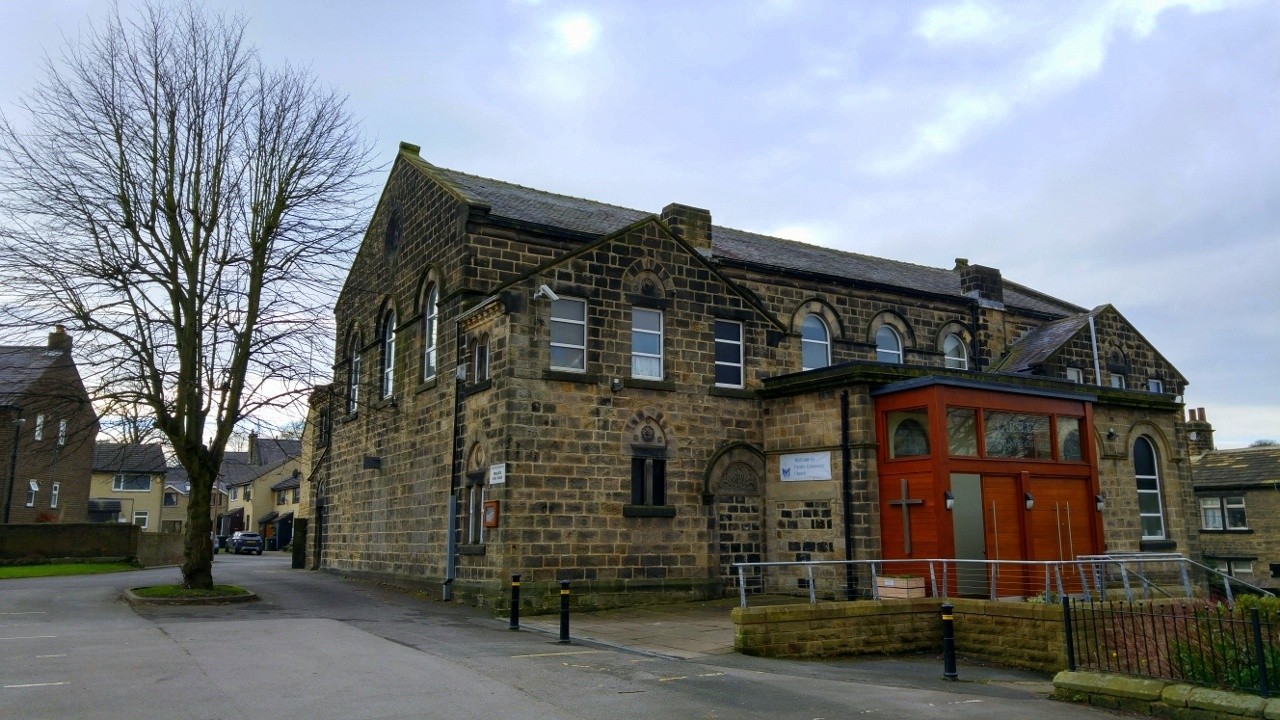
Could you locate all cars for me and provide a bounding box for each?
[211,533,219,554]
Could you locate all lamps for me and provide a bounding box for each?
[1095,494,1104,512]
[496,291,514,314]
[944,490,954,510]
[1024,491,1034,510]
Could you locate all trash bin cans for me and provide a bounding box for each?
[265,538,276,551]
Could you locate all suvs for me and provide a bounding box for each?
[224,530,263,556]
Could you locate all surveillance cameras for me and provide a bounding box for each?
[540,285,559,301]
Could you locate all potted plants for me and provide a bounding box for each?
[1106,427,1118,440]
[876,572,926,602]
